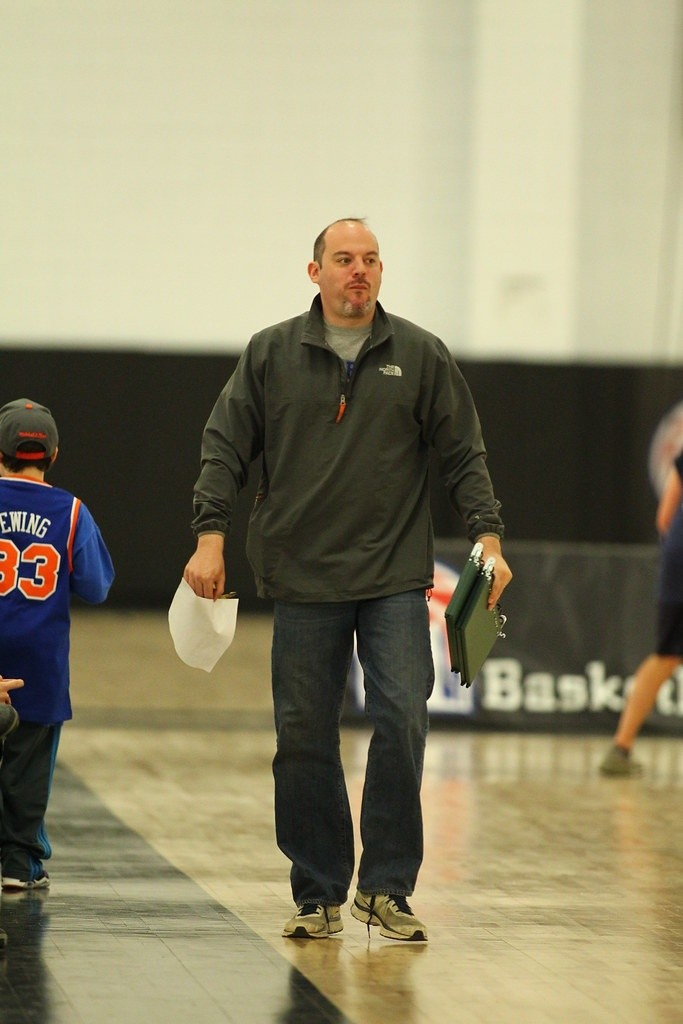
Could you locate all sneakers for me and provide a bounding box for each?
[599,747,647,777]
[280,904,345,938]
[349,889,429,942]
[1,869,51,889]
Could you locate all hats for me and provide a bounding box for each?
[0,397,59,460]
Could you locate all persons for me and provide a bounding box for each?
[599,450,683,776]
[0,397,118,896]
[182,215,511,949]
[0,671,25,763]
